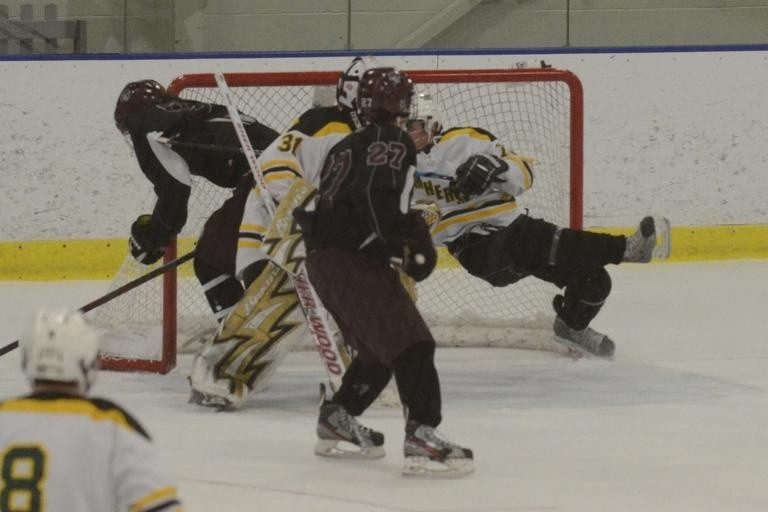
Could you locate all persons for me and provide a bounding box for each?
[303,68,477,476]
[0,306,186,512]
[114,79,280,322]
[190,57,417,410]
[403,91,672,361]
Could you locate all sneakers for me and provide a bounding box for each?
[318,402,384,445]
[404,422,472,459]
[554,316,615,357]
[622,217,658,262]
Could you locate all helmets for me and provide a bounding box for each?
[23,307,102,384]
[115,80,165,153]
[336,56,441,152]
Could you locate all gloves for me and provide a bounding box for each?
[453,154,508,194]
[129,214,167,262]
[409,229,436,282]
